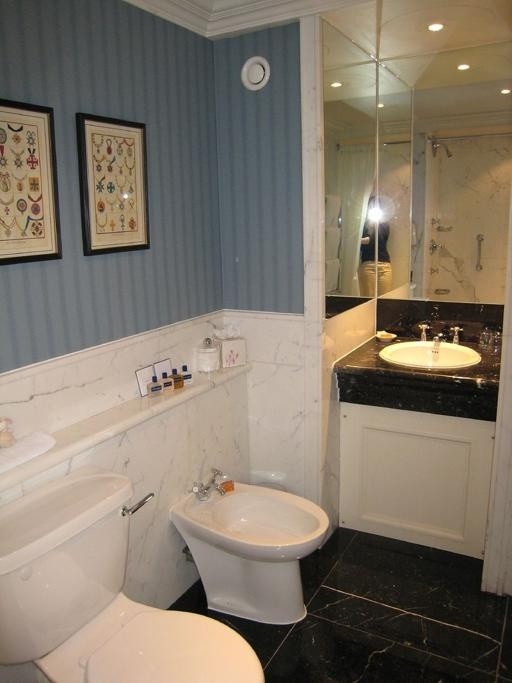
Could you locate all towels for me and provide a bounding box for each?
[0,430,56,476]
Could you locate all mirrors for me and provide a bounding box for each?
[319,1,512,308]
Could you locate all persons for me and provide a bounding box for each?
[360,196,392,297]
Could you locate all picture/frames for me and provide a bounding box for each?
[75,112,151,257]
[1,98,63,265]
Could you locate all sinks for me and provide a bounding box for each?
[378,341,482,372]
[170,478,329,563]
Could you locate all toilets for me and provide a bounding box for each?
[0,589,265,683]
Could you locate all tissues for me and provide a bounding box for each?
[214,322,248,367]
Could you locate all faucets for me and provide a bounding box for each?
[432,333,443,353]
[193,468,225,501]
[477,234,484,242]
